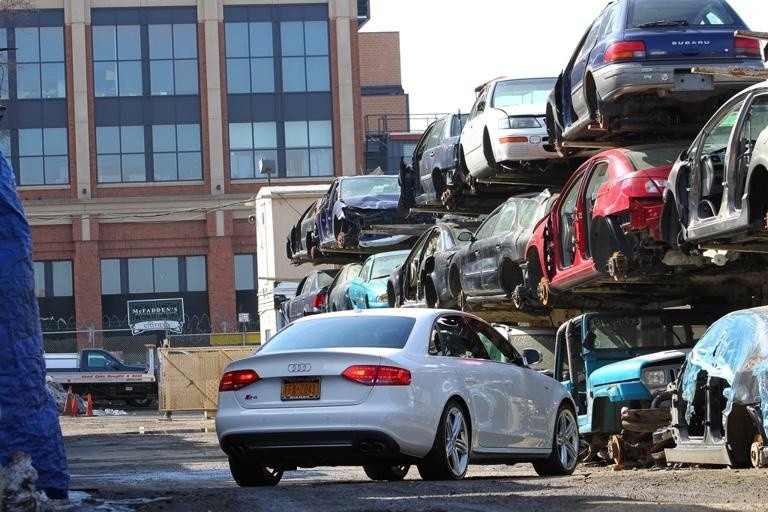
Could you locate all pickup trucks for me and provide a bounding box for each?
[46,347,157,407]
[540,303,704,461]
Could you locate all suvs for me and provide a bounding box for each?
[542,0,768,157]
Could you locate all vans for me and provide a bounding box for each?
[42,350,111,368]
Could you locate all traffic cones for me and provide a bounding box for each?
[72,392,81,417]
[55,386,73,416]
[81,392,98,417]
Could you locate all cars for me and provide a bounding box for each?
[656,73,767,252]
[522,137,699,310]
[455,75,559,186]
[284,174,435,264]
[214,303,581,485]
[281,267,342,322]
[396,113,511,213]
[446,187,561,312]
[318,262,363,312]
[344,248,412,310]
[385,219,480,309]
